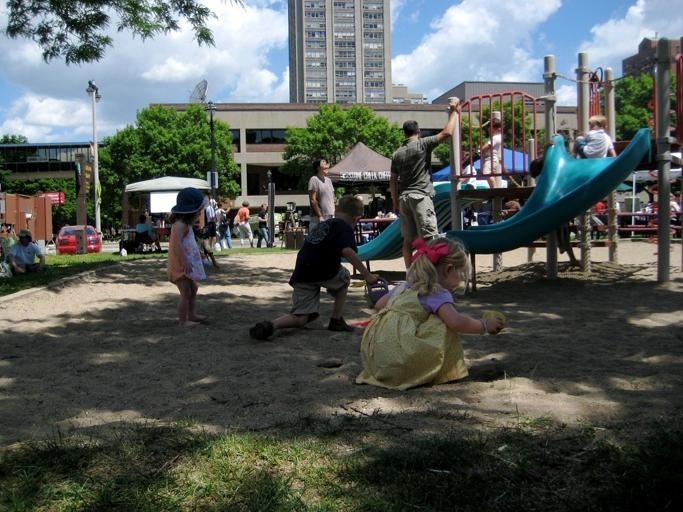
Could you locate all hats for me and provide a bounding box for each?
[16,229,32,237]
[172,187,209,214]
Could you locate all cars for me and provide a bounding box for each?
[54,225,100,253]
[237,213,281,239]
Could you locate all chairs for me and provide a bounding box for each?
[134,231,157,251]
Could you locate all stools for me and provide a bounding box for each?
[354,231,378,244]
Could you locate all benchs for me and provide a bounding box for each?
[591,210,683,238]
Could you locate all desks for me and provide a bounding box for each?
[358,218,396,245]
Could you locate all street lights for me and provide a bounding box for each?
[204,100,217,201]
[85,78,102,249]
[266,170,276,247]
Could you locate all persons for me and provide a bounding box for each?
[386,96,460,278]
[245,195,379,340]
[308,155,334,232]
[459,149,476,189]
[351,236,505,391]
[136,200,271,252]
[571,114,618,161]
[166,187,209,328]
[478,118,505,189]
[591,202,606,241]
[6,229,45,275]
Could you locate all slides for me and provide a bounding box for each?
[346,181,513,271]
[438,126,651,254]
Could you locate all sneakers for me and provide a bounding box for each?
[248,320,274,340]
[328,317,354,332]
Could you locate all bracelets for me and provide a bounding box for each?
[479,319,487,333]
